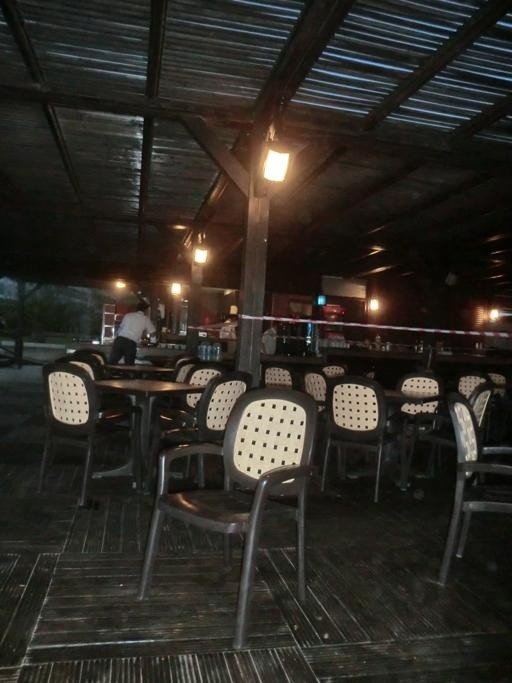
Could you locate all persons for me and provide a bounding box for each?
[107,300,156,366]
[218,314,238,340]
[260,320,276,352]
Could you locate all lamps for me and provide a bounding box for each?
[191,220,211,265]
[260,101,293,184]
[317,295,326,306]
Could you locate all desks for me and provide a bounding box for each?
[319,346,512,389]
[106,365,177,437]
[259,355,326,390]
[347,389,442,488]
[89,379,208,494]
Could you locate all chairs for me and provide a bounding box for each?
[486,372,509,442]
[135,388,317,649]
[263,364,295,390]
[56,355,127,488]
[174,353,198,371]
[158,362,225,480]
[302,371,330,474]
[319,377,407,503]
[392,374,442,485]
[37,363,134,507]
[321,362,347,380]
[438,392,512,585]
[157,360,201,413]
[147,370,252,496]
[403,380,496,492]
[75,349,108,378]
[455,371,493,445]
